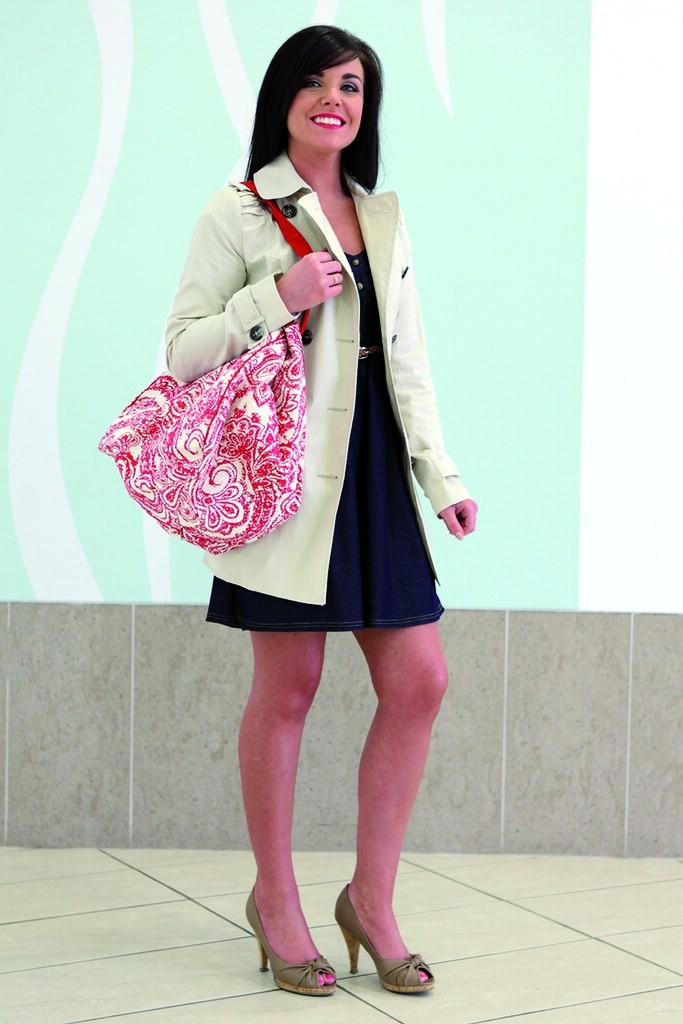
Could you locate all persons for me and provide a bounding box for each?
[165,25,478,995]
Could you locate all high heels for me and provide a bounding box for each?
[335,883,435,993]
[246,884,337,996]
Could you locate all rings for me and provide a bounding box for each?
[333,275,337,286]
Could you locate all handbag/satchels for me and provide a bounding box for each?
[97,181,319,557]
